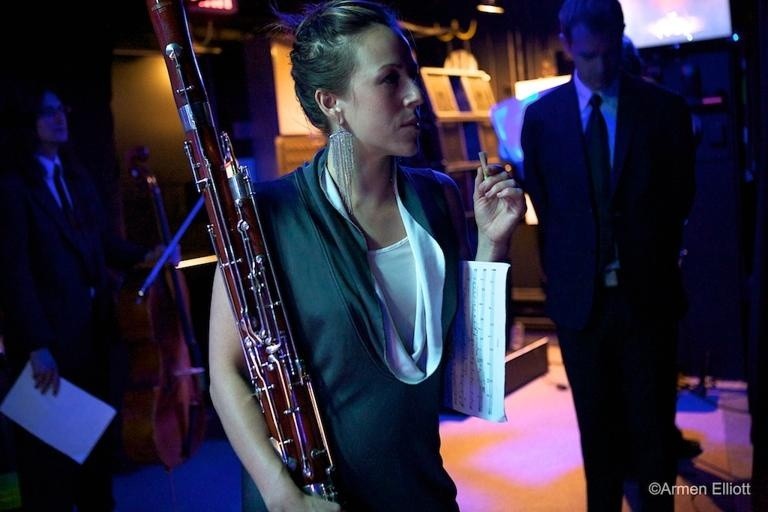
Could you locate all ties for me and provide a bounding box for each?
[584,94,609,272]
[54,164,77,232]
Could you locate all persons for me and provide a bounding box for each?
[518,0,698,512]
[1,80,182,512]
[207,0,531,512]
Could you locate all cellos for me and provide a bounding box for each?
[116,144,204,470]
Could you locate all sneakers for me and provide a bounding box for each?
[670,432,700,461]
